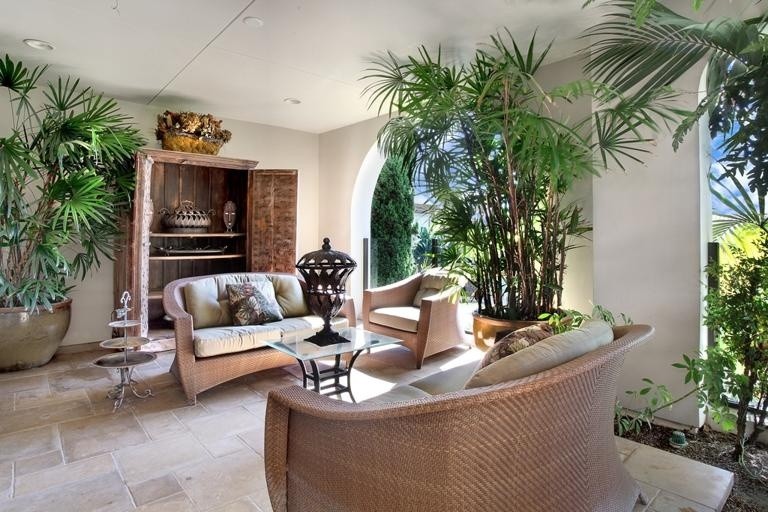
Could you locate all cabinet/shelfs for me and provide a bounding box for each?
[114,149,298,351]
[91,292,158,414]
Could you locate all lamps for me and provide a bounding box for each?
[293,238,358,348]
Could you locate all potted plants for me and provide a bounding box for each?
[356,24,694,352]
[0,54,149,372]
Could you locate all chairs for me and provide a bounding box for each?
[265,325,656,512]
[362,269,471,370]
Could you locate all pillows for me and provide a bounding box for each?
[413,273,455,307]
[463,319,616,389]
[471,324,553,374]
[226,282,283,326]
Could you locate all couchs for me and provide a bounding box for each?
[163,272,356,405]
